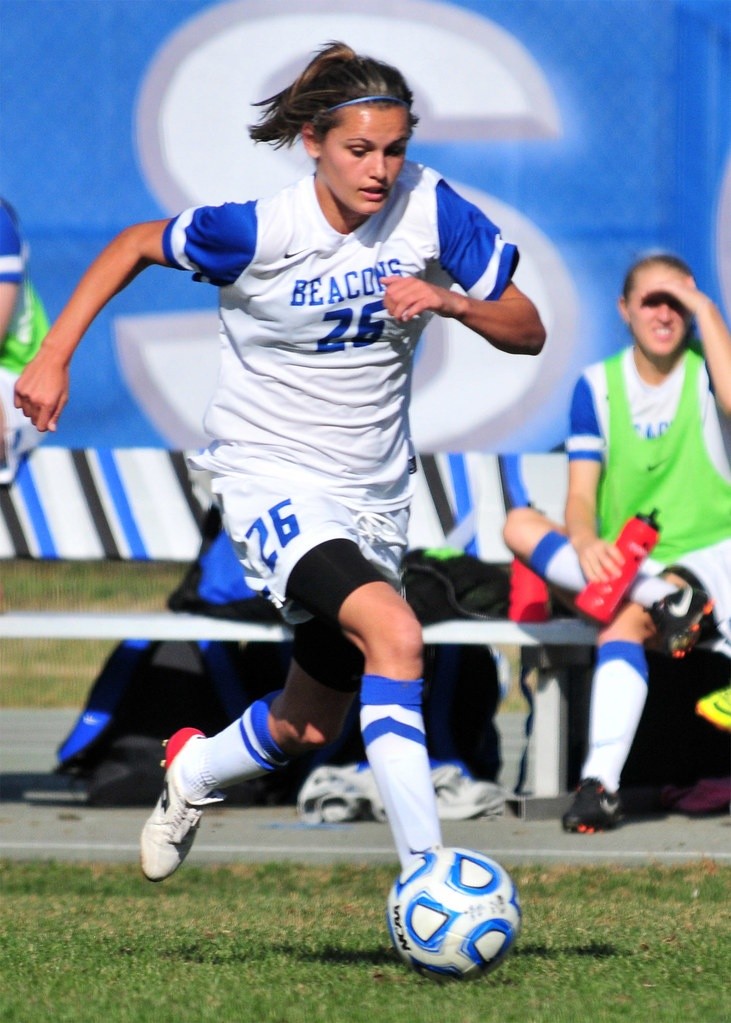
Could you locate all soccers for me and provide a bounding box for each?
[383,841,526,986]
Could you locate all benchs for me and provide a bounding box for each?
[2,446,607,815]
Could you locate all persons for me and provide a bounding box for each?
[15,35,550,903]
[3,190,64,514]
[506,250,731,834]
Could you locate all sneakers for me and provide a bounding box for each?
[137,727,227,882]
[647,588,714,663]
[561,776,627,833]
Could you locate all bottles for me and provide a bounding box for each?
[508,501,550,623]
[574,508,663,626]
